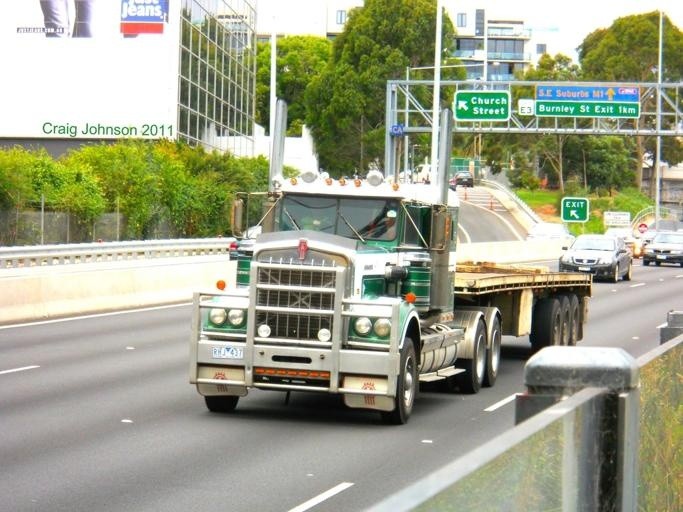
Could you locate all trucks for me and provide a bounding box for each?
[191,99,593,424]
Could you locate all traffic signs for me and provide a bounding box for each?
[561,198,588,222]
[532,87,639,103]
[454,93,509,122]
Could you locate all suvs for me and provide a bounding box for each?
[455,171,473,187]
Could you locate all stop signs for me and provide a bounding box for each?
[638,224,647,233]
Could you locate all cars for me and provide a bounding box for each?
[607,224,641,257]
[555,233,633,286]
[644,230,683,267]
[409,164,437,184]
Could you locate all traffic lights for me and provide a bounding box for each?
[535,100,639,119]
